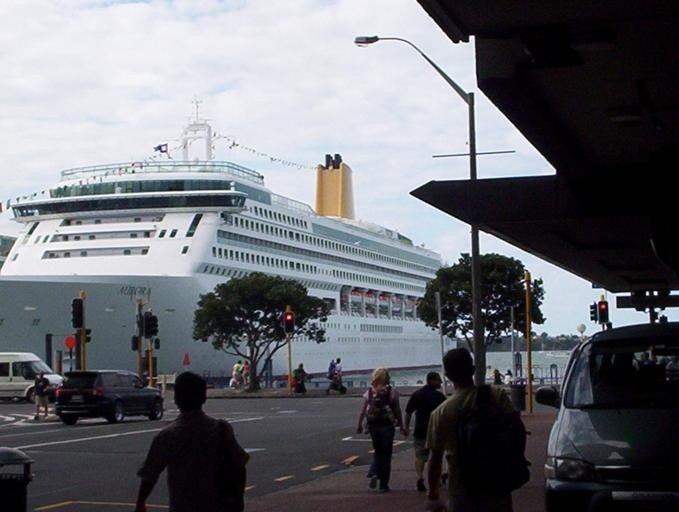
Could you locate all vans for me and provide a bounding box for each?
[535,322,678,511]
[0,351,66,402]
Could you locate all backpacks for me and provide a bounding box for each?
[454,384,530,499]
[364,385,395,433]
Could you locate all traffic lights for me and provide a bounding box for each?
[590,303,597,322]
[132,335,138,351]
[72,298,84,326]
[146,312,159,338]
[285,312,294,333]
[84,329,91,342]
[598,301,610,324]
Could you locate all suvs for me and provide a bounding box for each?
[53,370,165,424]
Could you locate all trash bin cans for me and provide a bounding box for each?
[510,379,528,411]
[0,446,35,512]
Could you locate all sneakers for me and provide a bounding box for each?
[417,478,427,492]
[379,483,393,490]
[369,475,377,488]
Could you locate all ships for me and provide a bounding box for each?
[0,93,458,387]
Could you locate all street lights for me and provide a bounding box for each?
[577,323,586,342]
[355,34,488,389]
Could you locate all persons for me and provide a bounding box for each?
[33,371,49,419]
[136,372,249,511]
[238,360,250,385]
[504,369,513,385]
[326,357,342,395]
[427,348,526,511]
[493,370,504,384]
[357,367,408,492]
[294,363,308,392]
[230,360,241,387]
[633,349,679,369]
[403,371,447,491]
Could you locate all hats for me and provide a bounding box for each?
[443,348,472,379]
